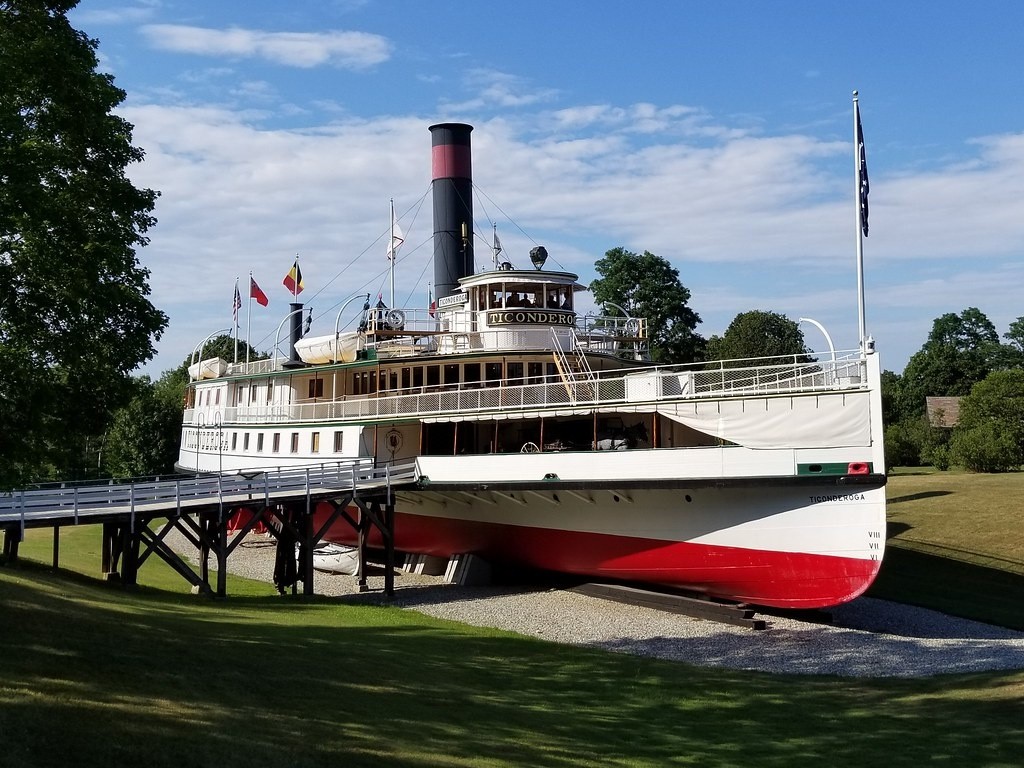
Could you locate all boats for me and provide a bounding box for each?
[294,330,368,363]
[190,355,229,380]
[170,122,890,607]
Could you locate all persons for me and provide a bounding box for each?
[546,294,557,308]
[561,292,571,310]
[519,293,531,307]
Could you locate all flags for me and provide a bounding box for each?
[493,228,501,265]
[429,290,436,318]
[251,276,268,306]
[233,283,242,329]
[857,104,870,236]
[386,202,403,265]
[282,260,305,295]
